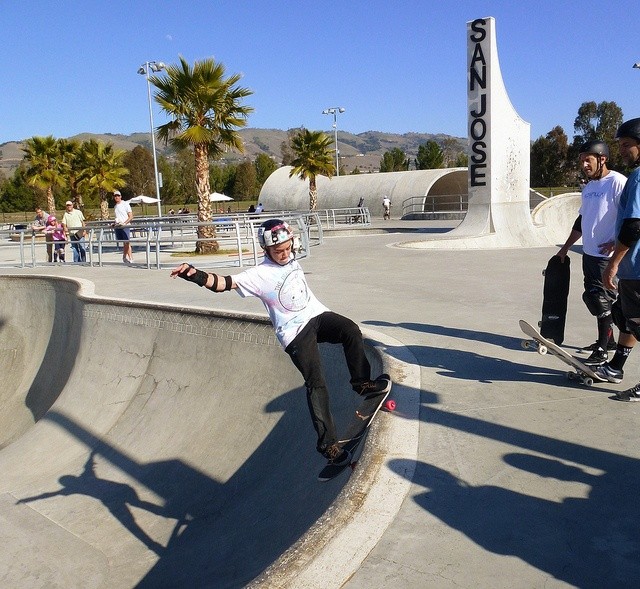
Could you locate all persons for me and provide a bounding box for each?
[112,190,135,264]
[41,215,67,262]
[248,204,256,219]
[31,207,55,261]
[168,207,190,223]
[254,202,264,219]
[167,218,392,465]
[555,138,628,365]
[589,117,640,402]
[60,200,88,263]
[382,195,392,215]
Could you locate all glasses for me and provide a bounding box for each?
[36,210,42,213]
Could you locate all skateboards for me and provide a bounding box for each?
[318,374,396,483]
[519,320,609,386]
[74,223,93,240]
[538,256,570,345]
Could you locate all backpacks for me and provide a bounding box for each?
[118,222,125,226]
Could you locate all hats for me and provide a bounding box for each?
[114,191,120,196]
[66,201,72,205]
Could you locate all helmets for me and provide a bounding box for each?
[384,196,388,198]
[615,118,640,139]
[259,219,294,247]
[579,139,609,162]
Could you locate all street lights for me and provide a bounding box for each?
[136,59,167,218]
[322,107,345,177]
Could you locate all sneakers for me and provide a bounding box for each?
[124,259,133,266]
[324,443,352,466]
[593,365,623,384]
[616,384,640,402]
[360,379,389,396]
[584,348,608,365]
[580,341,617,353]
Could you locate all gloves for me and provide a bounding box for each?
[178,262,208,287]
